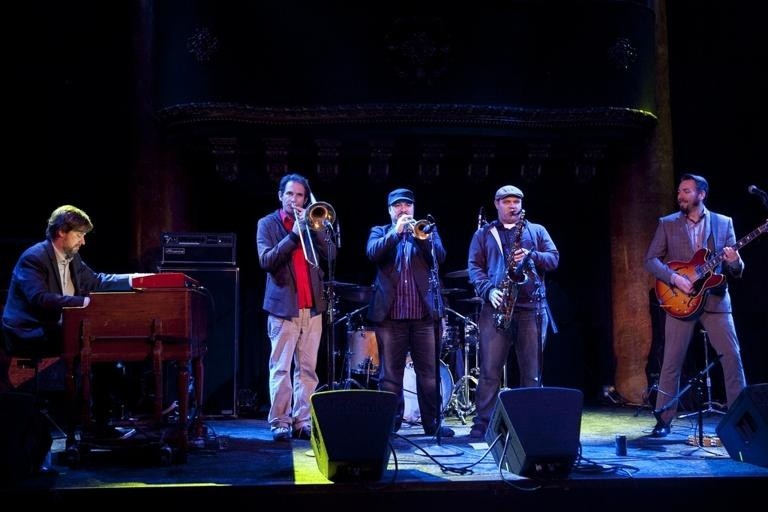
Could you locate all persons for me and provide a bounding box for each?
[254,175,338,440]
[3,205,159,440]
[366,187,454,439]
[468,185,561,438]
[644,174,746,437]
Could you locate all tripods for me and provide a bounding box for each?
[677,333,728,418]
[454,345,479,409]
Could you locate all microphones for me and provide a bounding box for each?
[516,246,534,275]
[477,206,485,229]
[748,185,767,204]
[324,219,338,237]
[422,222,438,234]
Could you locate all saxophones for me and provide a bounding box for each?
[492,209,534,336]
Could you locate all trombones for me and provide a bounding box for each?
[290,189,335,268]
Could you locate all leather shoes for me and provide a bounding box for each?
[273,427,290,442]
[470,424,483,438]
[292,424,311,441]
[652,424,670,438]
[424,426,455,437]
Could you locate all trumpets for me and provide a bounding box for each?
[400,214,430,240]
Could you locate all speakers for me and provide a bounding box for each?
[715,383,768,470]
[484,387,584,483]
[310,389,398,483]
[146,264,240,420]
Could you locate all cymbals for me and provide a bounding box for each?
[323,280,357,287]
[446,268,471,280]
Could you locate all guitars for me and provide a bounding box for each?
[654,219,767,320]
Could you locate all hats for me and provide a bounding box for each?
[388,188,415,205]
[495,185,524,201]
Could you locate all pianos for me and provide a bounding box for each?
[60,273,211,463]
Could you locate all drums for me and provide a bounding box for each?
[443,315,479,349]
[346,327,381,376]
[402,350,454,425]
